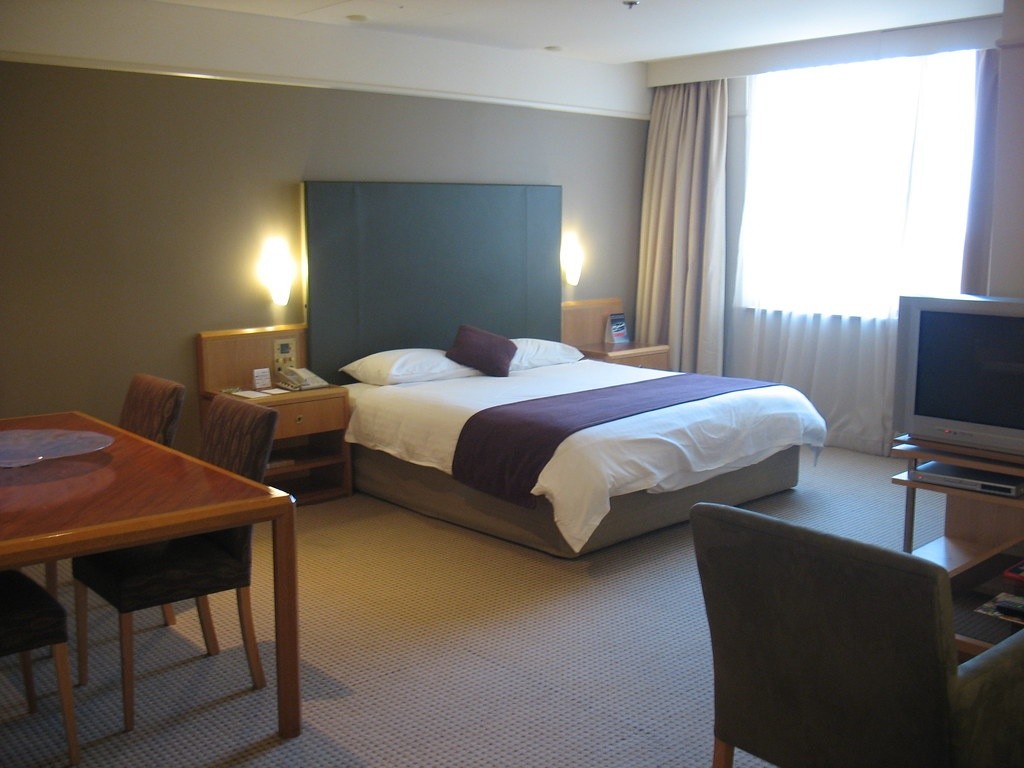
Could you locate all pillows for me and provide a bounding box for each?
[336,348,486,386]
[504,338,586,373]
[444,324,518,379]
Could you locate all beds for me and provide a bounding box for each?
[194,179,827,562]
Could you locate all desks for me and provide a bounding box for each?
[888,434,1024,553]
[1,409,302,740]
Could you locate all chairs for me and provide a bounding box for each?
[690,501,1024,768]
[45,369,189,599]
[69,394,279,736]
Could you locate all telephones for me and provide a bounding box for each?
[278,366,329,391]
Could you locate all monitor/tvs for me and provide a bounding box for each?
[893,294,1024,457]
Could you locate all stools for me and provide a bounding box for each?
[0,567,81,768]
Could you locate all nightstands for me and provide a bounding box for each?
[574,342,670,371]
[203,381,351,514]
